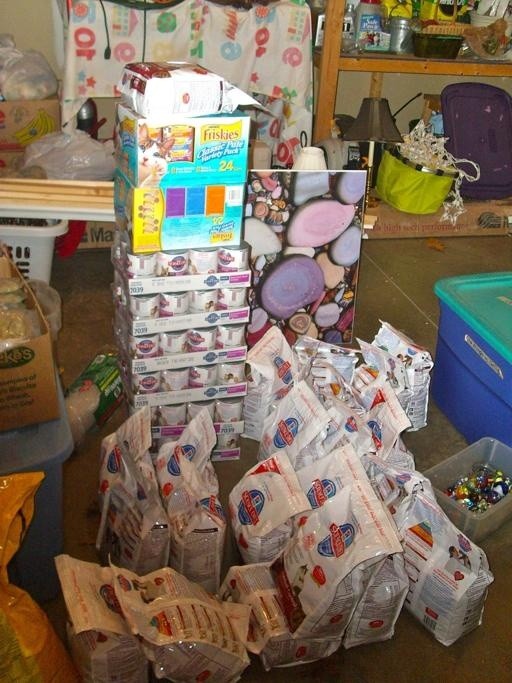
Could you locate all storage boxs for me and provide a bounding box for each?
[77,219,117,251]
[432,265,511,457]
[116,105,249,188]
[115,168,246,255]
[422,436,511,542]
[0,93,60,152]
[413,33,463,59]
[0,247,61,434]
[0,362,74,609]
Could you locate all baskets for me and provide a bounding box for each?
[418,0,474,35]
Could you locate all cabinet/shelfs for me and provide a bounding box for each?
[311,1,512,238]
[0,150,116,229]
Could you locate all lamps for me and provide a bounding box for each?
[343,96,403,207]
